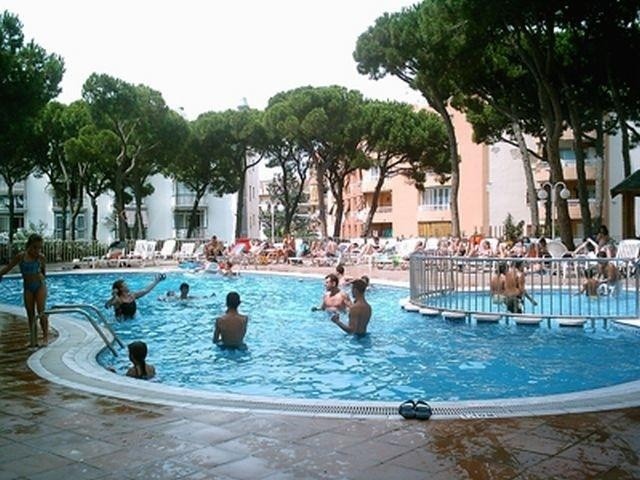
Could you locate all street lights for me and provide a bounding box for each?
[537,181,571,241]
[261,199,287,245]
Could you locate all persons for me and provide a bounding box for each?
[157,283,196,301]
[576,226,621,300]
[108,340,155,380]
[307,264,372,337]
[212,292,249,351]
[0,234,48,347]
[486,259,538,314]
[432,224,551,274]
[204,223,425,278]
[105,273,167,322]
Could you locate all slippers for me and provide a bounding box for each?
[398,400,432,420]
